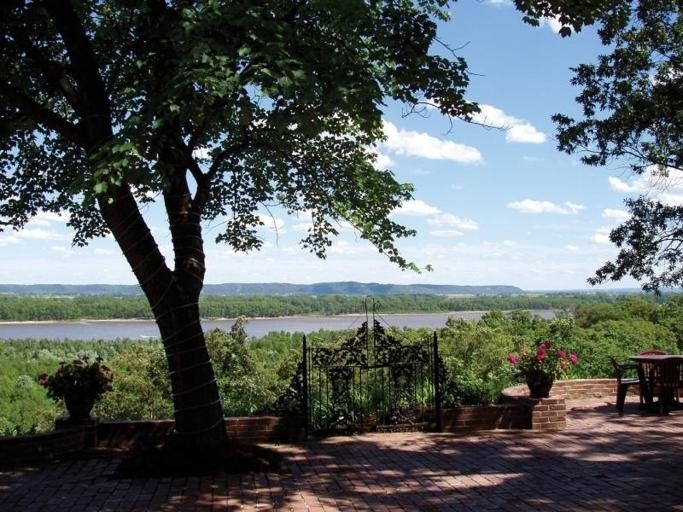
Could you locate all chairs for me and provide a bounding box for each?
[608,351,683,414]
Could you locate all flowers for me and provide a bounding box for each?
[37,354,114,402]
[507,340,578,383]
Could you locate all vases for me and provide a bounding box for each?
[524,372,555,398]
[63,385,95,419]
